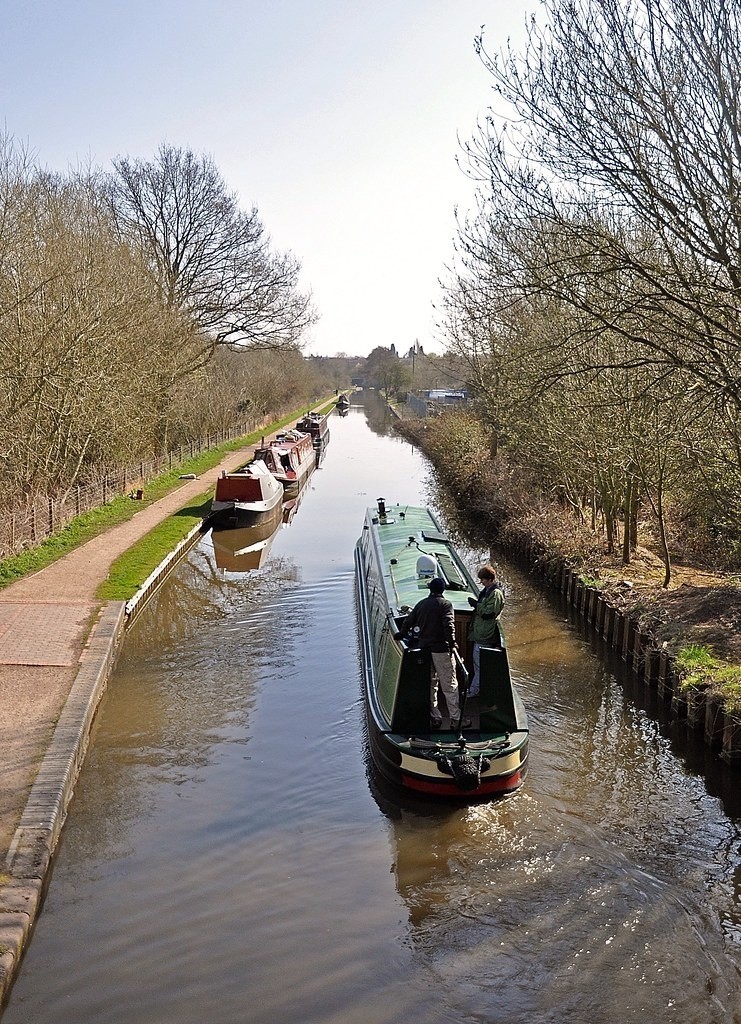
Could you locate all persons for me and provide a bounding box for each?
[461,568,506,698]
[401,577,472,730]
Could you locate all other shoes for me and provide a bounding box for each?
[432,718,441,727]
[451,718,471,728]
[462,689,480,698]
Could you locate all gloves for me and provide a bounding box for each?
[467,597,477,608]
[393,632,409,641]
[450,641,460,650]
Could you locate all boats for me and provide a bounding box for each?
[336,393,350,409]
[355,497,530,803]
[211,410,330,528]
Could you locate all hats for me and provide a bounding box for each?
[427,578,444,590]
